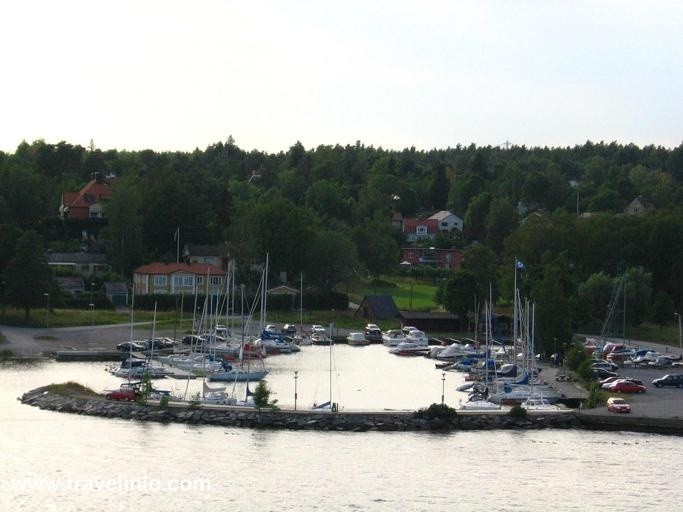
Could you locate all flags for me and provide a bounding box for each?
[516,260,526,271]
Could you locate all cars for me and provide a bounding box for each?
[605,396,630,413]
[650,374,682,388]
[105,389,136,402]
[582,359,645,393]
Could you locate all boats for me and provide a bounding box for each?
[584,267,682,365]
[364,323,383,343]
[383,326,431,354]
[310,322,334,344]
[346,332,370,345]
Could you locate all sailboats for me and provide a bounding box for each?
[429,258,560,411]
[119,300,178,399]
[200,345,243,405]
[112,251,312,381]
[311,323,344,412]
